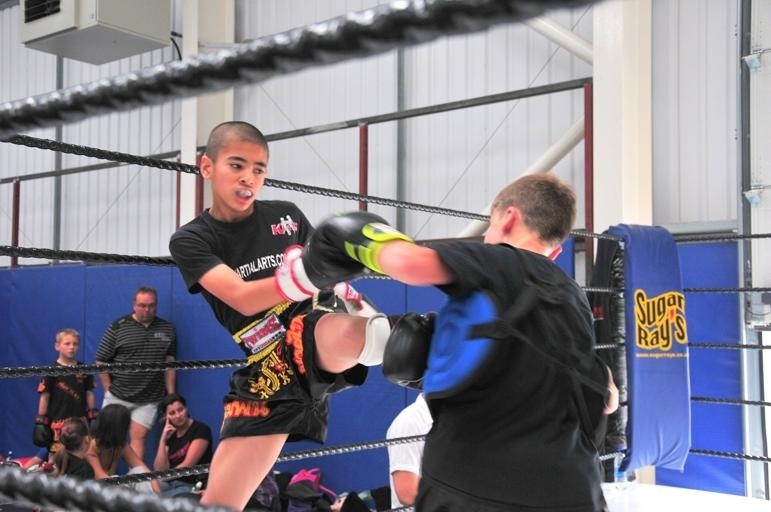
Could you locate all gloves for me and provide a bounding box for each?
[86,408,99,428]
[33,415,53,447]
[383,310,437,392]
[275,212,415,303]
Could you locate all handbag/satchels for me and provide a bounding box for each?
[287,469,319,492]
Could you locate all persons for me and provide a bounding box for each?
[169,121,403,511]
[313,174,620,511]
[35,328,101,466]
[387,393,436,511]
[86,403,162,494]
[95,288,177,462]
[52,417,95,480]
[154,393,213,497]
